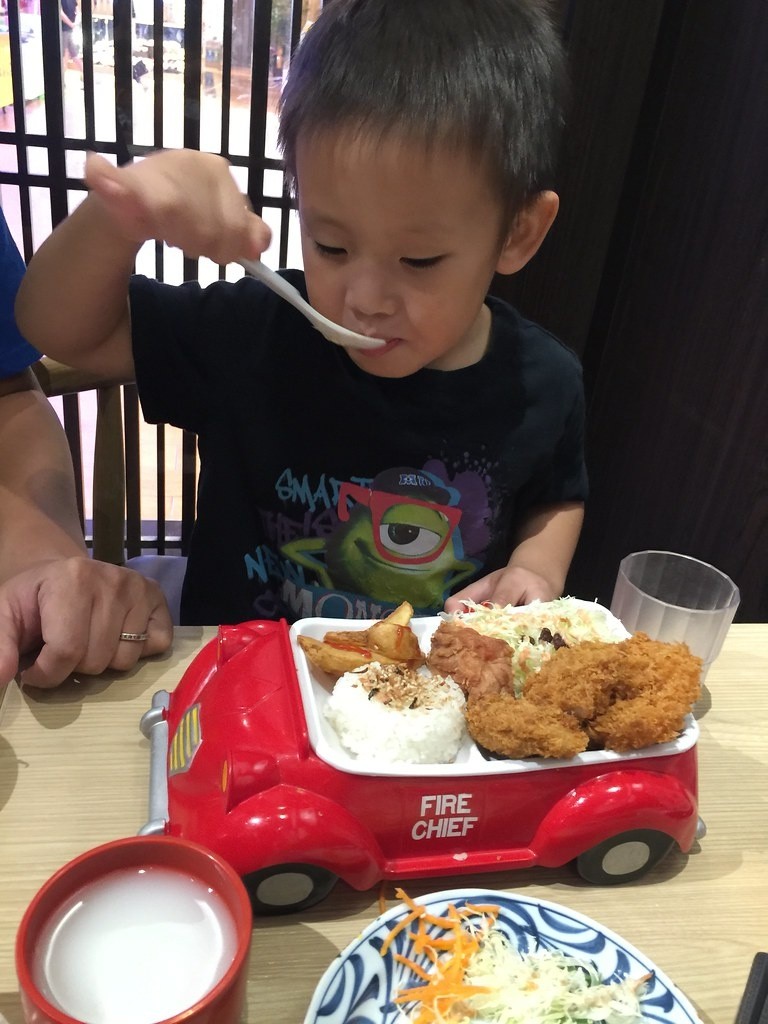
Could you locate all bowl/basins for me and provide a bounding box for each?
[14,834,252,1024]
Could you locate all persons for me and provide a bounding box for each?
[60,0,85,90]
[13,0,591,624]
[0,209,171,689]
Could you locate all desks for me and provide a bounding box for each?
[0,625,768,1024]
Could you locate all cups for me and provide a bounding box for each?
[609,550,741,708]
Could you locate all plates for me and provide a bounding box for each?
[302,887,705,1024]
[287,599,700,778]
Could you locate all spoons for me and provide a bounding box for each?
[237,257,386,350]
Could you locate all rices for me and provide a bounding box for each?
[322,660,468,766]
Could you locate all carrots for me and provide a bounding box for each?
[378,881,501,1024]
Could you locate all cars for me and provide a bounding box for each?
[139,593,709,919]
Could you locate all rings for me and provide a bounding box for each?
[121,632,148,641]
